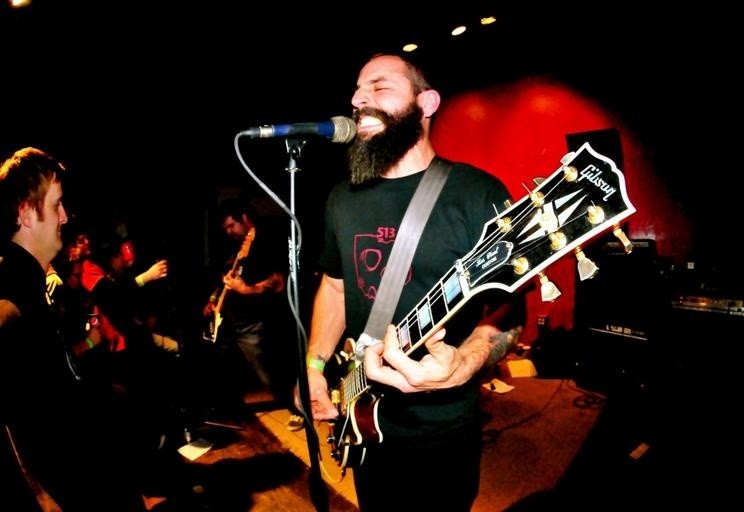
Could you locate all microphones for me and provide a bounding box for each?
[243,114,359,148]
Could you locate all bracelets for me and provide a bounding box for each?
[137,275,145,286]
[305,355,324,371]
[86,337,93,348]
[208,294,218,300]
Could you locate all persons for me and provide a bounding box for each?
[0,143,146,510]
[286,46,541,512]
[201,197,308,433]
[42,213,192,482]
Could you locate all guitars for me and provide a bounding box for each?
[204,228,257,342]
[323,142,639,468]
